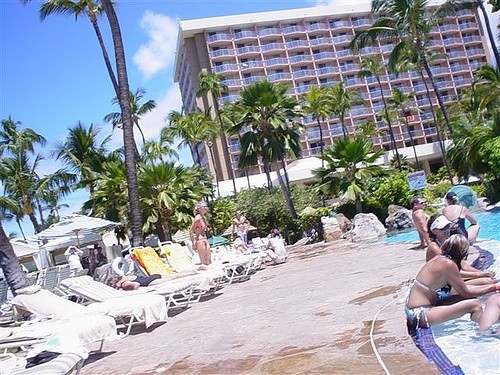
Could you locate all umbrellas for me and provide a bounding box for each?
[9,214,120,259]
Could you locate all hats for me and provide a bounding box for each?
[445,192,457,200]
[430,215,453,231]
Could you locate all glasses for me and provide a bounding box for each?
[420,202,424,204]
[440,223,450,231]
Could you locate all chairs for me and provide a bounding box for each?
[0,242,267,375]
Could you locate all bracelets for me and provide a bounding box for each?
[494,285,496,292]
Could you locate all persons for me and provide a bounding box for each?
[64,246,84,273]
[479,294,500,330]
[84,247,107,274]
[110,268,197,289]
[265,229,287,265]
[232,210,250,244]
[411,192,498,283]
[190,201,211,264]
[233,236,261,254]
[406,234,500,328]
